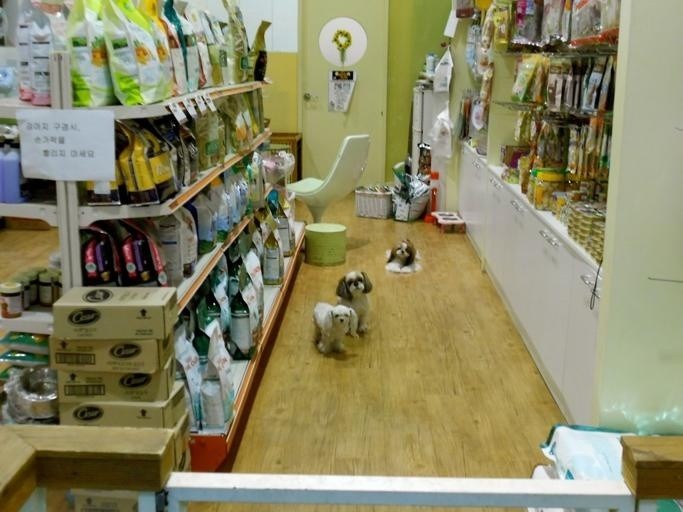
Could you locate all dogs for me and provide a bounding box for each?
[386,238,417,269]
[333,271,373,334]
[311,299,356,355]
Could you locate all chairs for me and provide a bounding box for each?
[287,134,374,222]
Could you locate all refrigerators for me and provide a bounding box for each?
[409,86,449,176]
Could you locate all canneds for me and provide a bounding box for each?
[0,253,64,318]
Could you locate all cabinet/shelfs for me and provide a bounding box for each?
[0,37,304,471]
[455,0,683,431]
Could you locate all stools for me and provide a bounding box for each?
[306,222,346,266]
